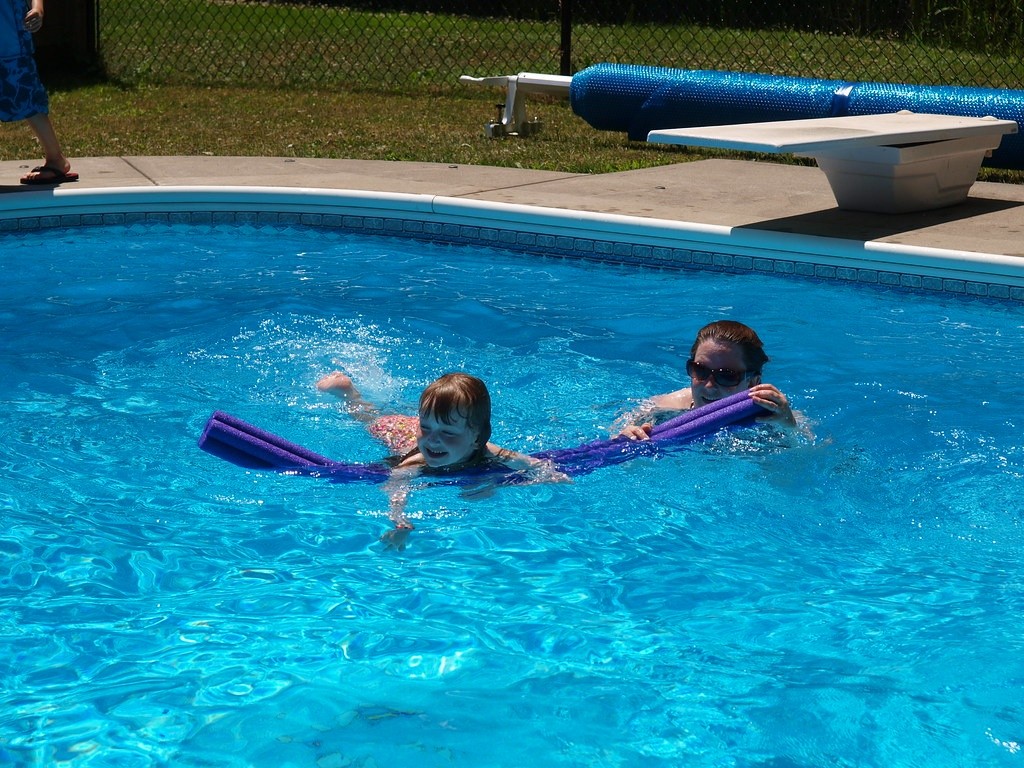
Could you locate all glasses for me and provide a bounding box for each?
[685,358,752,387]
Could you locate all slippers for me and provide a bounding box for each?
[20,166,79,185]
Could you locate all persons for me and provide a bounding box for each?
[0,0,79,183]
[609,320,813,442]
[317,372,514,476]
